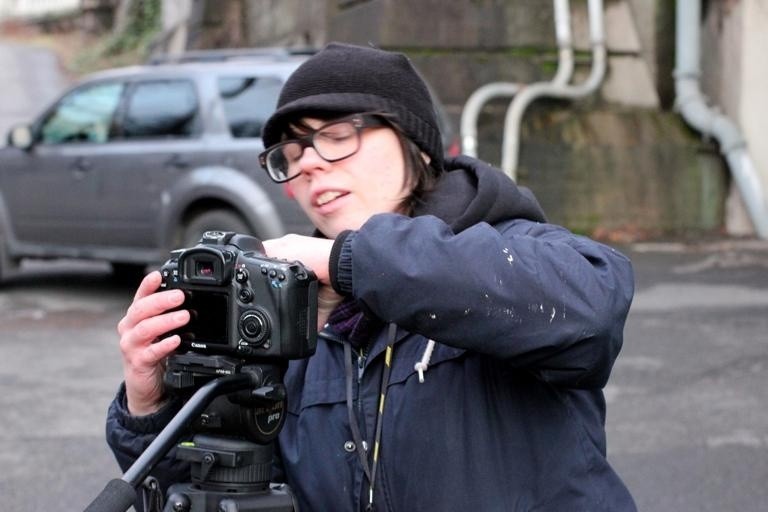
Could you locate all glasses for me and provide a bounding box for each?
[258,113,384,184]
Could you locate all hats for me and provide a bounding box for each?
[262,41,445,179]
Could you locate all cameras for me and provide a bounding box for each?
[155,226,318,389]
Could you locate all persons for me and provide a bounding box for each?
[103,41,638,511]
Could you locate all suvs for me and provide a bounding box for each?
[0,47,461,291]
